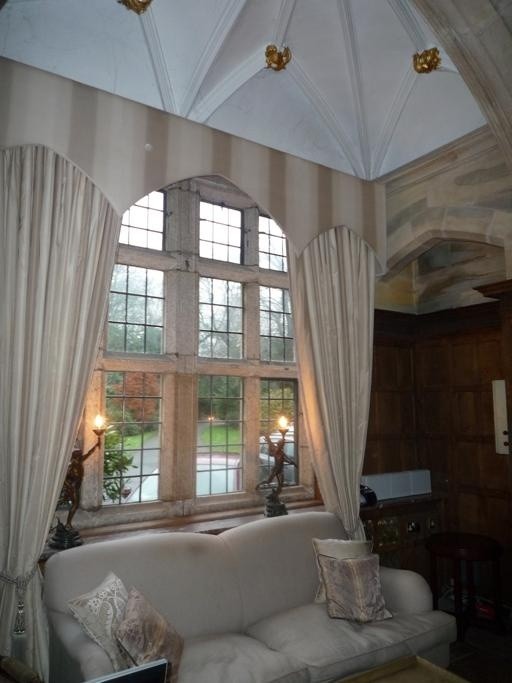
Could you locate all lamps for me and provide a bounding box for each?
[254,414,300,518]
[48,411,108,549]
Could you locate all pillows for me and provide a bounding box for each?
[64,570,186,682]
[312,535,395,625]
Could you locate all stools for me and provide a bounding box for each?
[423,532,510,642]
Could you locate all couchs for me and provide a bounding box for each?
[41,509,458,683]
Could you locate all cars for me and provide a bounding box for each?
[122,432,295,503]
